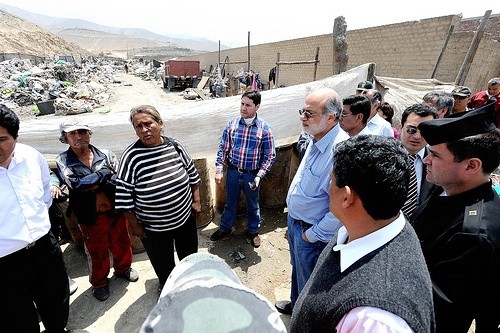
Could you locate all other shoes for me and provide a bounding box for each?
[248,232,263,247]
[275,300,294,316]
[210,227,232,241]
[156,284,163,301]
[114,267,140,282]
[92,285,110,301]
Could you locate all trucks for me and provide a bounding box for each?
[160,59,202,91]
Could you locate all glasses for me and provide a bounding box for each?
[298,109,325,119]
[403,126,424,134]
[341,112,357,119]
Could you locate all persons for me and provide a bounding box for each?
[210,89,276,247]
[114,105,201,293]
[138,252,287,333]
[288,134,437,333]
[406,103,500,333]
[341,77,500,220]
[0,103,70,333]
[56,115,140,301]
[274,86,350,316]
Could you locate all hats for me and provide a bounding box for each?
[58,116,92,133]
[139,253,288,333]
[450,86,472,98]
[356,81,374,91]
[417,100,497,147]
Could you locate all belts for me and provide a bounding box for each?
[228,162,259,174]
[294,217,314,229]
[0,233,50,258]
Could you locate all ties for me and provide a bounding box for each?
[401,153,419,220]
